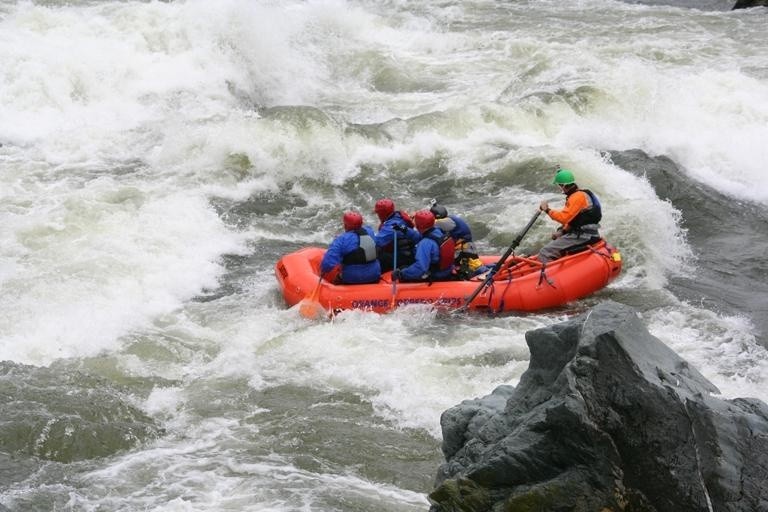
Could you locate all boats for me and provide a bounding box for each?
[270,239,622,314]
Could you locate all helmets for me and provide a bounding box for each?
[430,204,447,219]
[415,210,435,234]
[375,199,394,223]
[552,169,575,185]
[344,211,363,232]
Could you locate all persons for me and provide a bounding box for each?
[537,169,602,263]
[318,198,475,284]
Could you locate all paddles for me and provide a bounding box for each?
[450,206,543,313]
[299,275,322,320]
[384,226,397,314]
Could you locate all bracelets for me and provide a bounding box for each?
[544,209,551,213]
